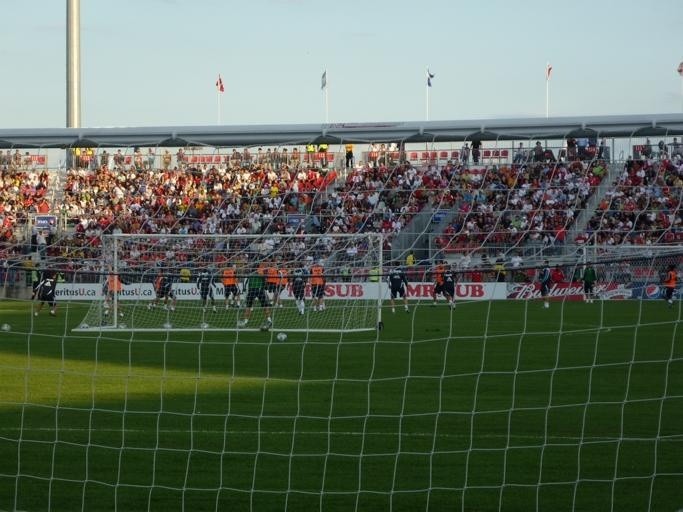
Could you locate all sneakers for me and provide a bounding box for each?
[49,312,56,317]
[406,304,456,313]
[239,321,271,328]
[668,301,678,308]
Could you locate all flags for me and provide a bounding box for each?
[426,71,434,88]
[544,64,553,80]
[319,71,327,90]
[213,77,226,95]
[676,60,683,78]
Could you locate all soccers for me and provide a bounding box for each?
[200,322,209,329]
[2,323,11,332]
[236,320,245,327]
[276,332,288,343]
[80,322,90,329]
[163,322,173,329]
[118,323,127,331]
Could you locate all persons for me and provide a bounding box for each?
[0,130,682,327]
